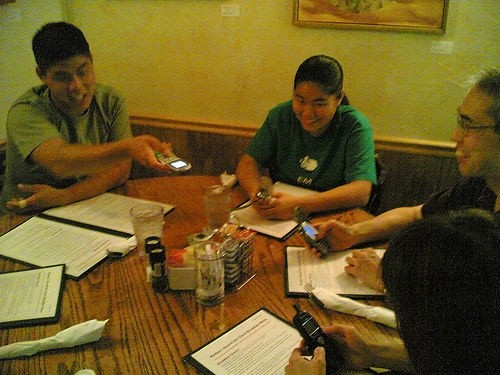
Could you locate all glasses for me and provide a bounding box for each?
[456,116,498,135]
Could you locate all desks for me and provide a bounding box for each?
[0,174,414,375]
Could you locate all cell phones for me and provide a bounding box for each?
[299,219,332,259]
[291,303,349,374]
[256,189,273,204]
[154,150,192,173]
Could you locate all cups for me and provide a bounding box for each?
[203,186,233,232]
[192,241,227,307]
[129,202,165,261]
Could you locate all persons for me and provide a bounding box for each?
[305,66,500,260]
[234,53,378,220]
[284,207,500,375]
[0,20,172,217]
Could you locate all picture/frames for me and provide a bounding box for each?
[292,0,449,36]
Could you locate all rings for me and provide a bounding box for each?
[19,200,27,208]
